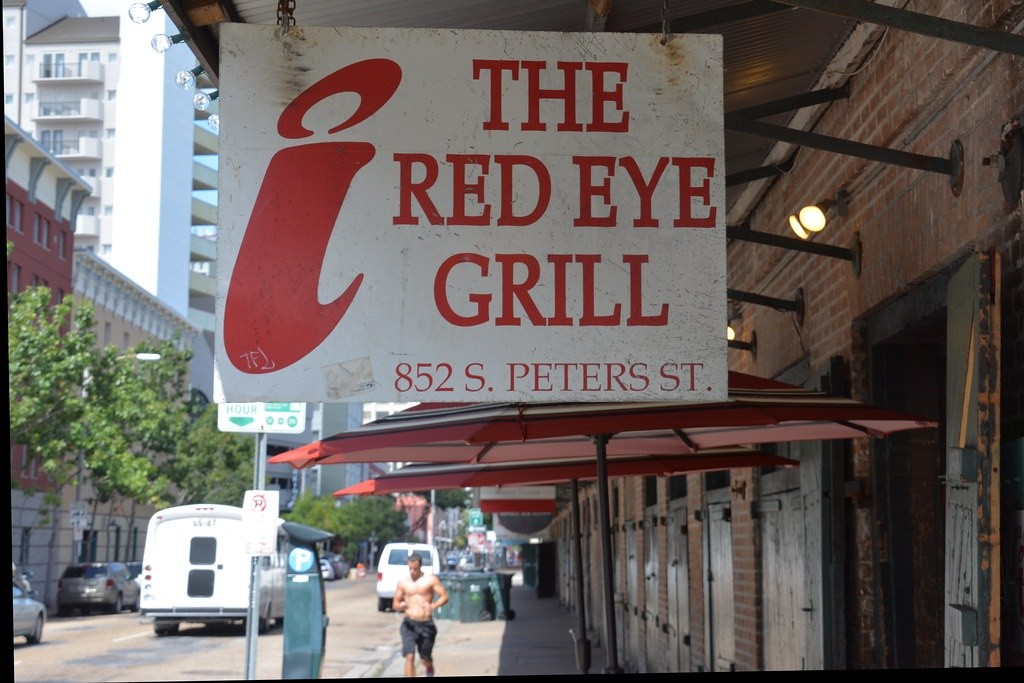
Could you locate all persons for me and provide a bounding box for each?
[392,553,448,677]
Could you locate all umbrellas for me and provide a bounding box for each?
[269,367,933,675]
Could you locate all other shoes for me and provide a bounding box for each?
[426,665,434,677]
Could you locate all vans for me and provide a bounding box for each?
[376,542,440,612]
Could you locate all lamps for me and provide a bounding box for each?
[726,306,742,340]
[788,190,852,241]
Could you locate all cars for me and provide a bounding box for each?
[13,563,34,592]
[325,554,349,579]
[13,582,47,645]
[443,550,501,571]
[320,559,335,581]
[57,563,140,615]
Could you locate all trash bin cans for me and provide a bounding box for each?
[434,571,516,623]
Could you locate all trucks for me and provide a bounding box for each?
[138,504,336,636]
